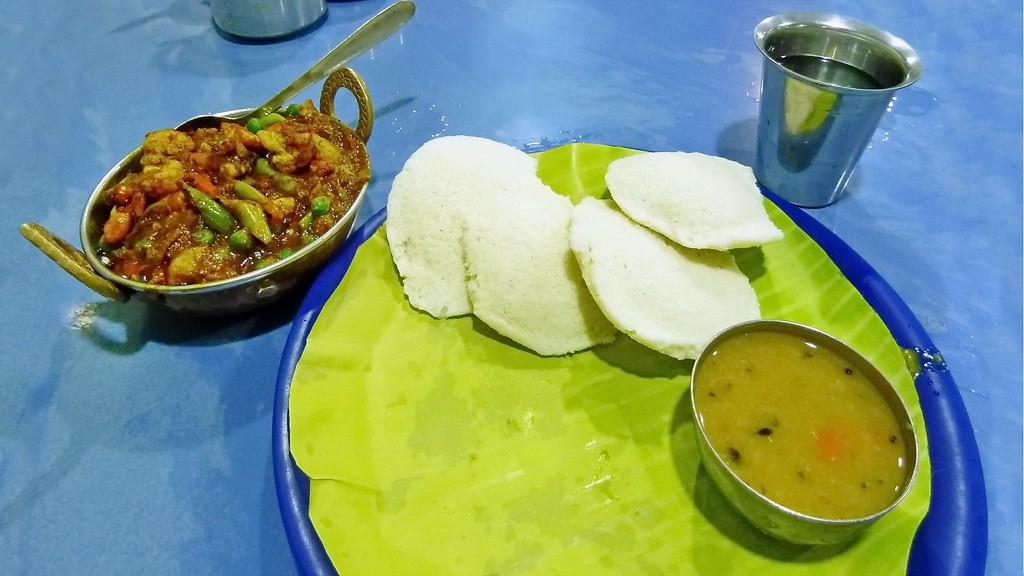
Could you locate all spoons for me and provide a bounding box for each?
[173,2,417,128]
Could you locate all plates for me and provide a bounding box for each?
[271,148,989,575]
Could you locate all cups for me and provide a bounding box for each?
[210,1,327,41]
[753,13,925,209]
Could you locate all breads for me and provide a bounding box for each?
[387,136,784,360]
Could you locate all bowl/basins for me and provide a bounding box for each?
[16,66,374,319]
[691,317,920,546]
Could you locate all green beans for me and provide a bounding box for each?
[98,104,331,287]
